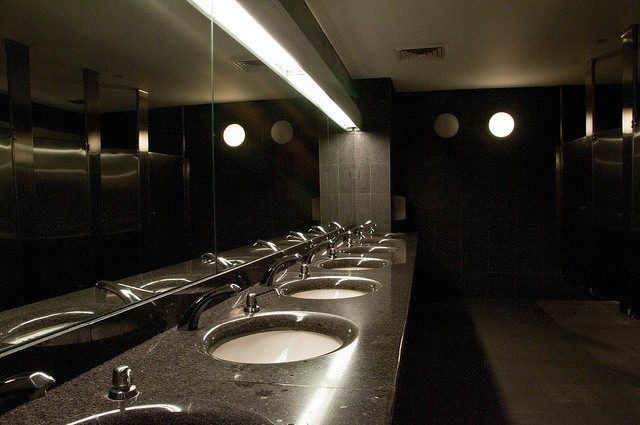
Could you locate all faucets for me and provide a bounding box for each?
[201,252,230,269]
[332,230,352,245]
[261,253,302,287]
[306,225,327,235]
[94,278,142,305]
[251,239,282,253]
[1,370,56,396]
[301,239,334,265]
[362,220,377,229]
[327,220,342,229]
[177,283,241,333]
[286,231,308,242]
[350,225,364,236]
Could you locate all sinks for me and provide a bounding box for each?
[68,403,261,425]
[140,279,189,292]
[375,232,402,237]
[345,245,390,255]
[9,309,96,338]
[227,259,245,268]
[276,275,378,300]
[360,239,391,247]
[202,310,358,364]
[320,258,387,271]
